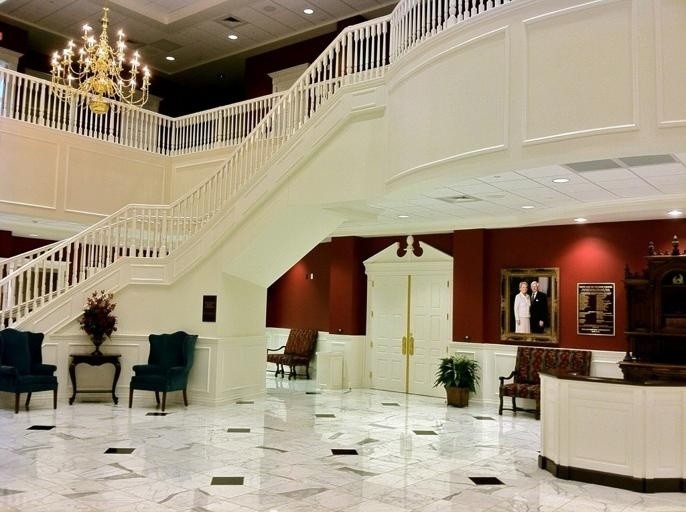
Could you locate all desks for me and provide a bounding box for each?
[68,354,121,405]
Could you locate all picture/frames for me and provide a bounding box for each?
[500,268,560,346]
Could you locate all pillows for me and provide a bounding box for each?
[151,358,177,375]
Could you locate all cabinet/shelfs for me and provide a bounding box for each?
[619,235,686,380]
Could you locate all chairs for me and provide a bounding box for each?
[0,328,59,413]
[267,328,318,380]
[129,331,199,411]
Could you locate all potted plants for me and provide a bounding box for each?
[431,353,481,407]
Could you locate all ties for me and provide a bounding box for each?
[531,294,534,303]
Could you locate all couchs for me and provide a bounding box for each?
[499,347,591,420]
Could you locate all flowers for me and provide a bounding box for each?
[78,290,118,339]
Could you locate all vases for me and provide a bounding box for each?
[91,337,105,356]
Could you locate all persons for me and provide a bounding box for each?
[514,282,531,333]
[530,281,548,333]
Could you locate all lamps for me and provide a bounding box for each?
[50,7,152,114]
